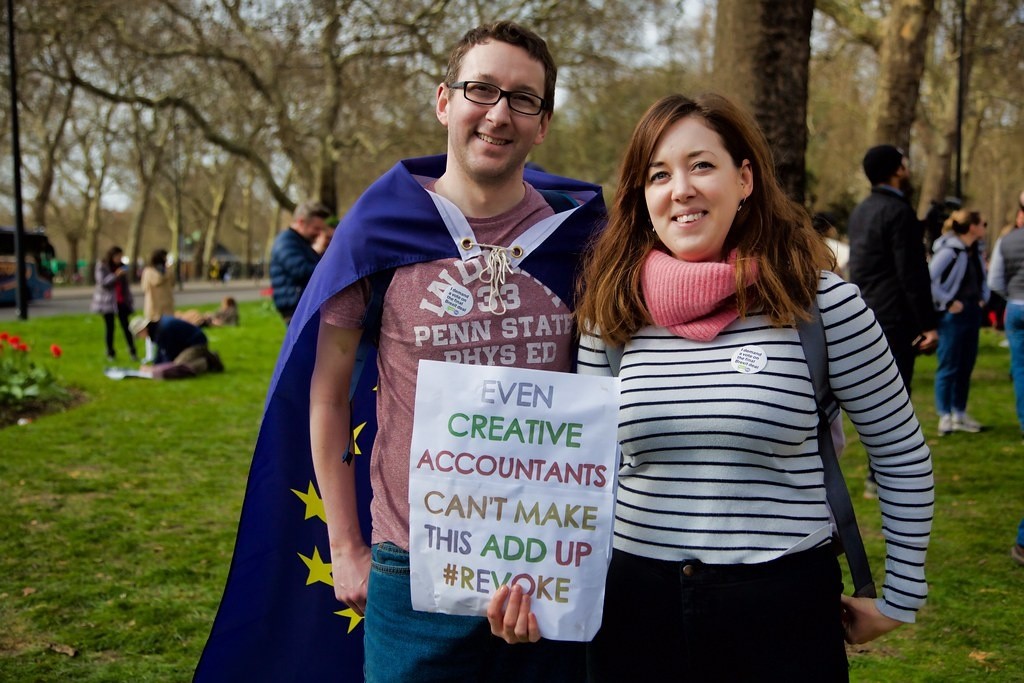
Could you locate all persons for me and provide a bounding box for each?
[485,94,934,683]
[812,143,1024,565]
[309,20,611,683]
[93,201,337,377]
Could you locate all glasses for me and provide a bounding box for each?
[448,81,547,115]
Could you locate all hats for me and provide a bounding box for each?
[130,315,151,336]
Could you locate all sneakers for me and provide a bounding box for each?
[937,415,952,437]
[952,418,979,433]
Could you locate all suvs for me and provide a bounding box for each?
[0,225,56,304]
